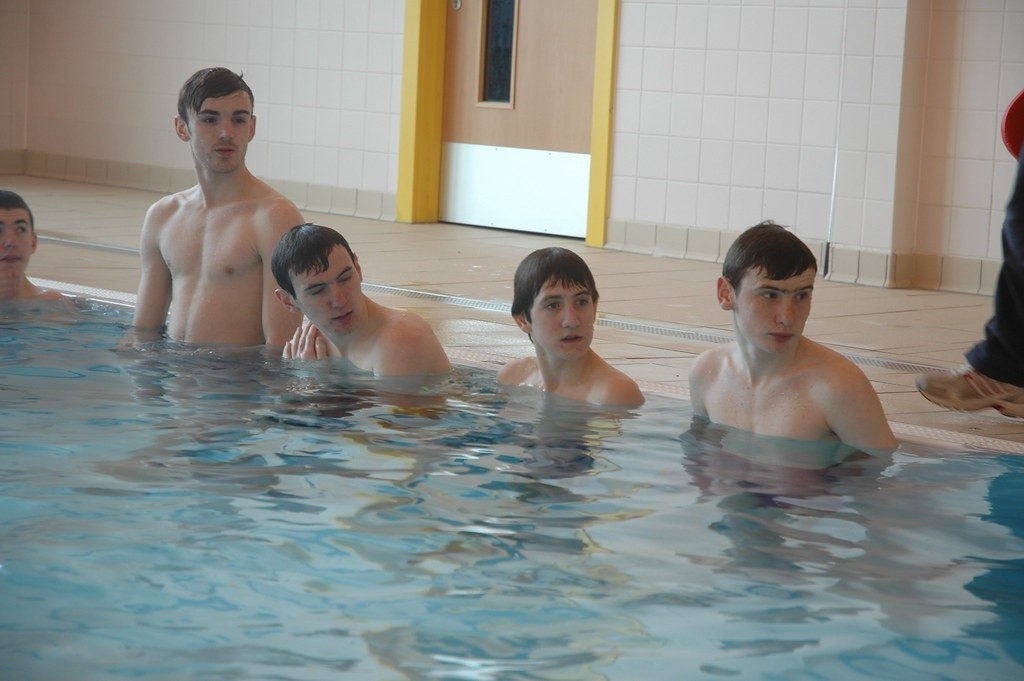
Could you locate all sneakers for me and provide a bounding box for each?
[915,365,1024,418]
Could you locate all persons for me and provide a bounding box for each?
[687,219,900,507]
[496,247,645,405]
[915,150,1024,419]
[123,67,303,360]
[270,222,454,410]
[0,190,65,301]
